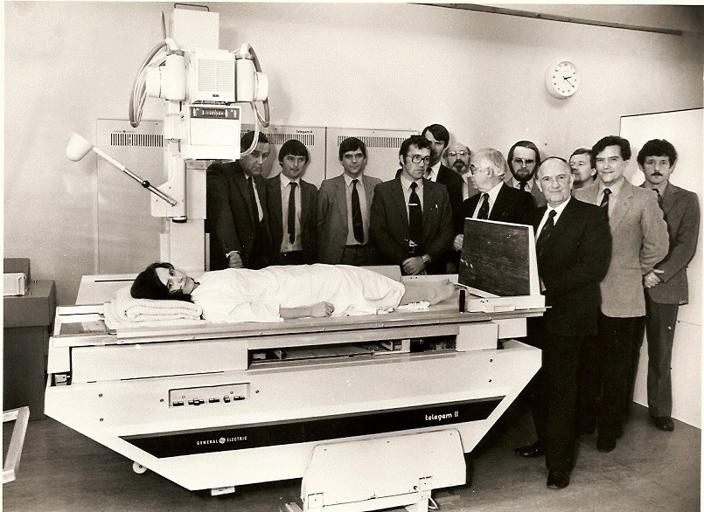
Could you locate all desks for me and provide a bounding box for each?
[3,277,57,422]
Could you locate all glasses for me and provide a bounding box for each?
[543,174,567,183]
[469,167,477,174]
[447,150,467,157]
[406,154,430,163]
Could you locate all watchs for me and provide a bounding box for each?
[420,254,431,265]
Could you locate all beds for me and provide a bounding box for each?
[43,264,549,512]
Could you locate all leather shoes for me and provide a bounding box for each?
[516,444,544,457]
[655,415,674,431]
[597,428,623,453]
[546,468,569,489]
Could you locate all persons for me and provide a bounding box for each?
[265,139,321,268]
[393,124,464,229]
[633,141,701,430]
[441,142,481,200]
[131,261,457,320]
[461,148,541,243]
[315,136,384,265]
[505,141,547,208]
[513,156,612,492]
[569,134,672,454]
[568,148,598,190]
[367,135,459,274]
[205,131,275,270]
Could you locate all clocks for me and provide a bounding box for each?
[544,57,582,99]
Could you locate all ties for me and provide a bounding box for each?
[351,179,364,243]
[289,182,297,244]
[519,182,526,190]
[248,176,259,222]
[408,182,423,239]
[600,189,612,213]
[477,193,489,219]
[536,210,557,259]
